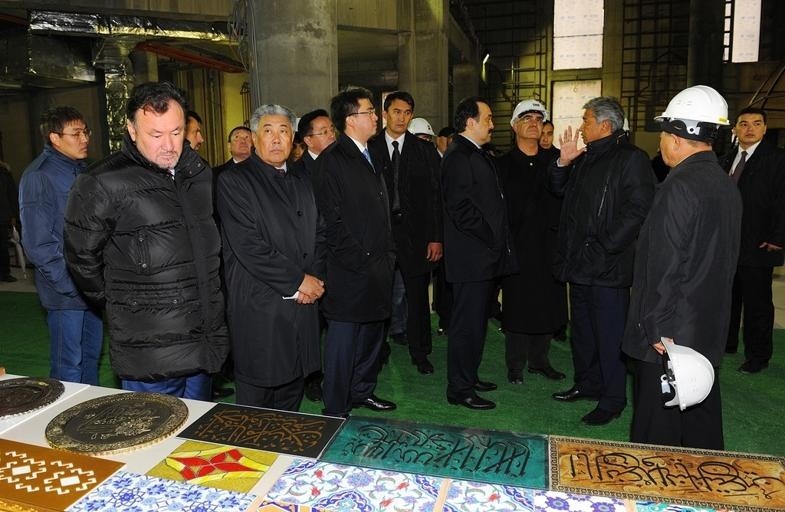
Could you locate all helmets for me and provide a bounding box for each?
[658,335,715,411]
[654,85,732,143]
[510,99,548,124]
[409,117,437,137]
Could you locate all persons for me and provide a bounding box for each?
[18,104,110,385]
[407,115,435,144]
[181,109,234,398]
[210,126,254,224]
[63,81,233,404]
[544,96,655,431]
[0,163,19,285]
[432,128,461,335]
[537,120,566,156]
[618,85,743,451]
[368,92,445,378]
[214,104,329,412]
[496,99,564,386]
[291,110,336,174]
[287,118,307,165]
[716,107,785,374]
[439,96,512,411]
[309,86,400,420]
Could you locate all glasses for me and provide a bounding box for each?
[307,124,334,136]
[344,108,376,118]
[48,128,91,137]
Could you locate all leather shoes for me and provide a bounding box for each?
[528,364,566,381]
[740,361,770,374]
[447,393,496,411]
[551,385,600,402]
[352,395,397,411]
[413,358,434,375]
[208,387,235,399]
[473,376,497,391]
[1,274,18,282]
[583,406,621,425]
[304,383,322,402]
[506,370,524,384]
